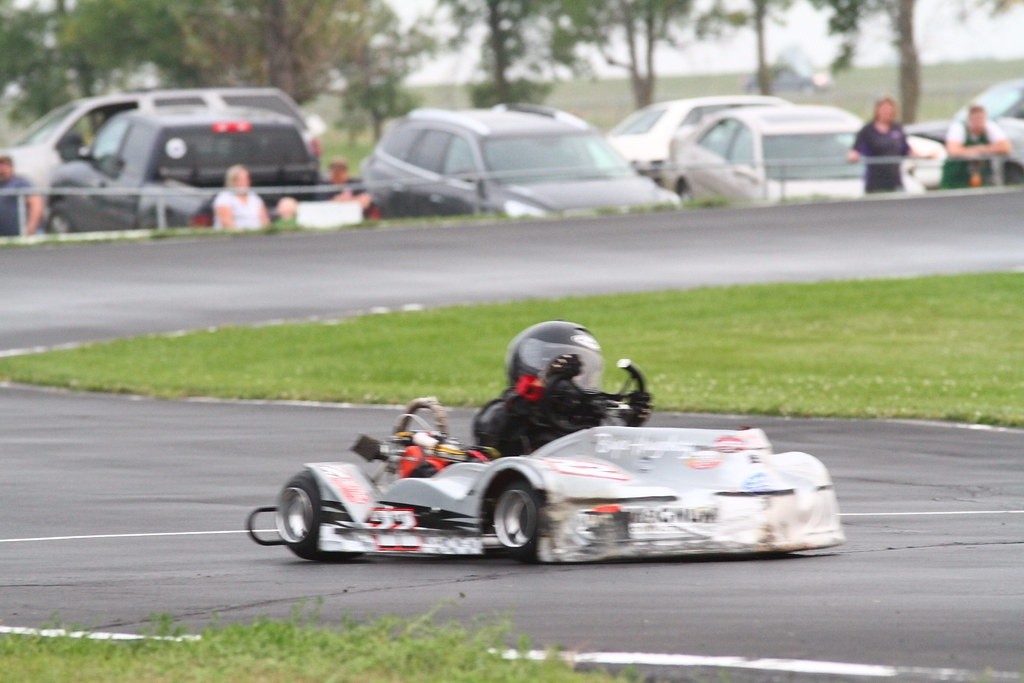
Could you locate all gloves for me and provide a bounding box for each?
[626,395,654,420]
[541,355,580,384]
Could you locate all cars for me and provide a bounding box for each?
[902,80,1023,185]
[672,104,928,194]
[605,93,794,181]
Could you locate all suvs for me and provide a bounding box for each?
[29,104,324,232]
[354,100,693,214]
[0,83,321,194]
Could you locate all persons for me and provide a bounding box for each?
[0,155,43,238]
[848,97,936,194]
[316,157,373,222]
[938,105,1011,187]
[211,163,297,233]
[475,321,655,456]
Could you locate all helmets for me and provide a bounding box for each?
[506,322,604,398]
